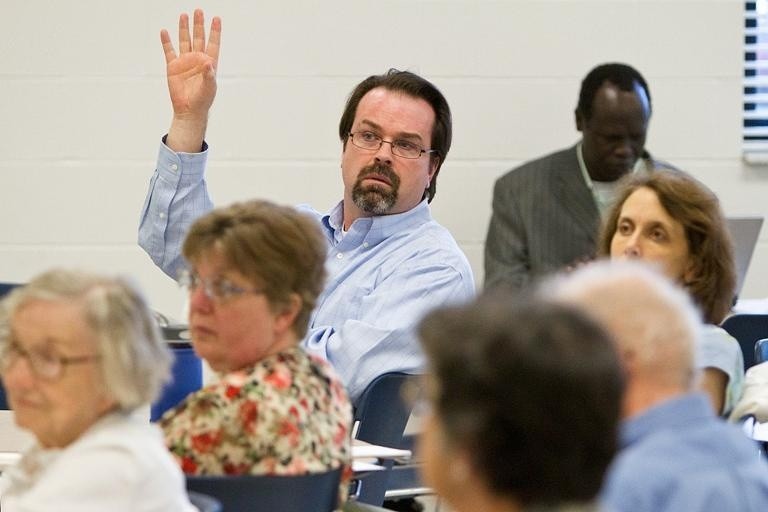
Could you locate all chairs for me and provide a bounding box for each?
[350,369,427,506]
[180,464,345,510]
[718,312,768,370]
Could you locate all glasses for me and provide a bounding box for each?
[0,334,103,377]
[346,127,437,159]
[186,269,263,300]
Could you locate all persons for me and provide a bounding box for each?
[595,169,748,425]
[477,60,717,315]
[525,258,766,512]
[0,265,200,511]
[406,291,624,509]
[164,197,357,495]
[134,6,478,418]
